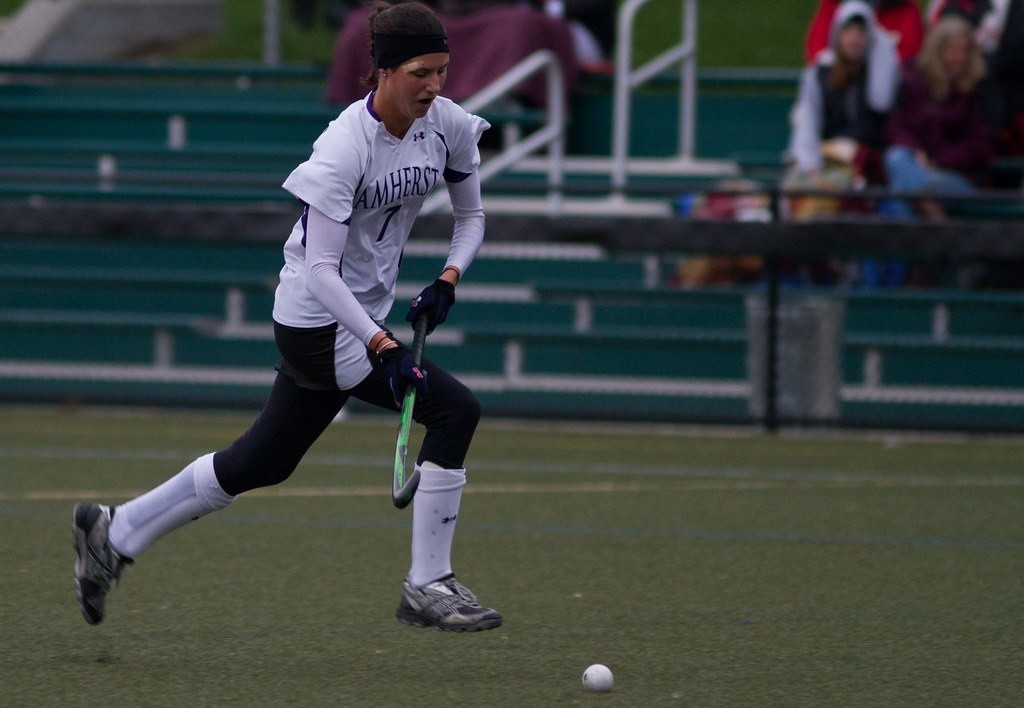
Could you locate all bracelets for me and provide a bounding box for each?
[440,268,459,287]
[377,340,395,353]
[374,336,389,350]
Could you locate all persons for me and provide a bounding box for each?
[324,0,601,156]
[72,0,504,631]
[778,0,1024,294]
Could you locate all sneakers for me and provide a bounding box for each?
[70,502,135,626]
[394,572,502,633]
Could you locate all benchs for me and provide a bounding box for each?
[0,58,1024,432]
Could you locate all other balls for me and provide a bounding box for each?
[582,662,614,693]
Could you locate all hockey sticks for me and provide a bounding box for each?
[390,311,431,511]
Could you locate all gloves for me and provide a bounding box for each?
[380,346,427,422]
[405,279,455,337]
